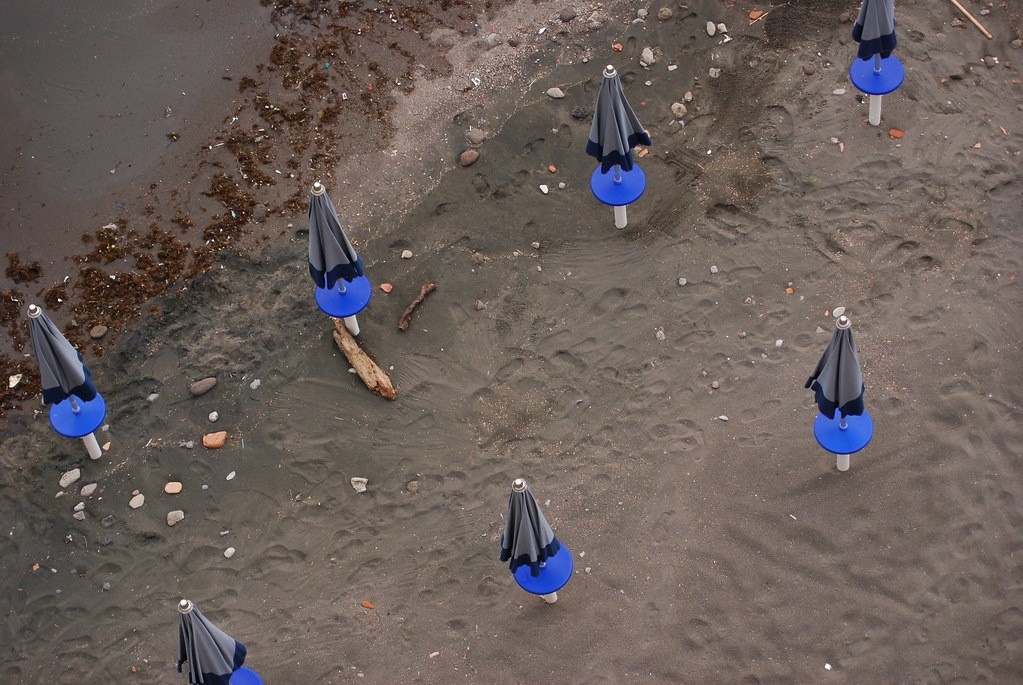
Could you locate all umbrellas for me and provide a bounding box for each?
[177,599,247,685]
[309,183,364,335]
[500,479,560,603]
[804,317,865,472]
[28,304,102,459]
[852,0,897,126]
[586,64,651,227]
